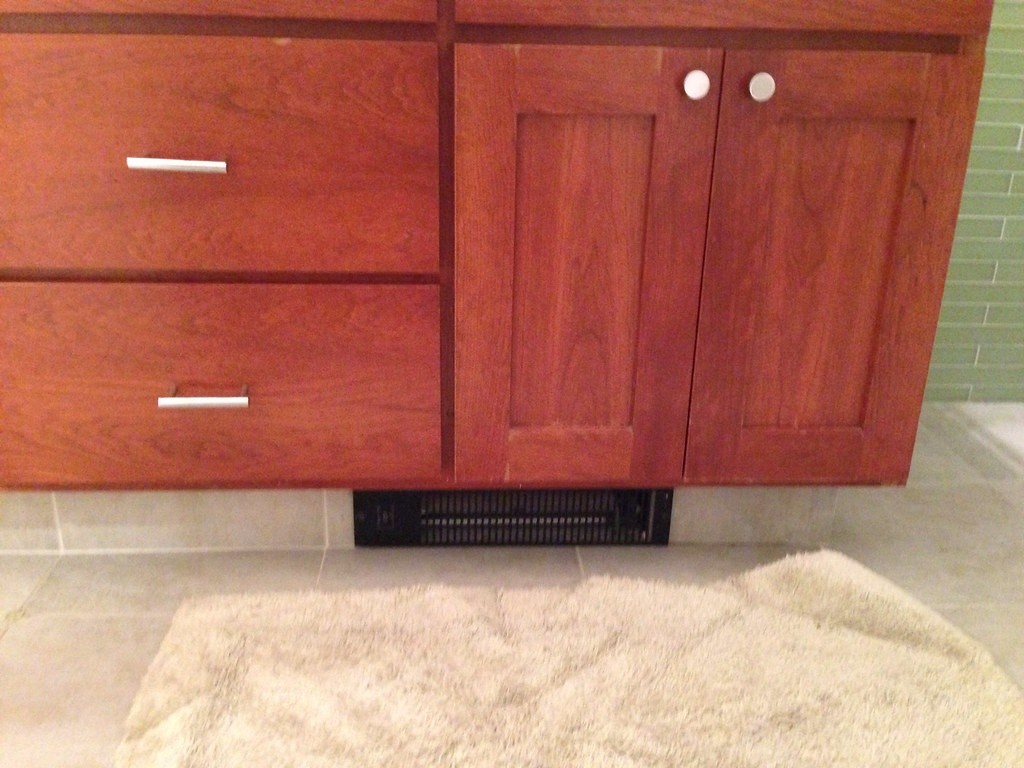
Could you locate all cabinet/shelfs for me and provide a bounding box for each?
[0,0,989,492]
